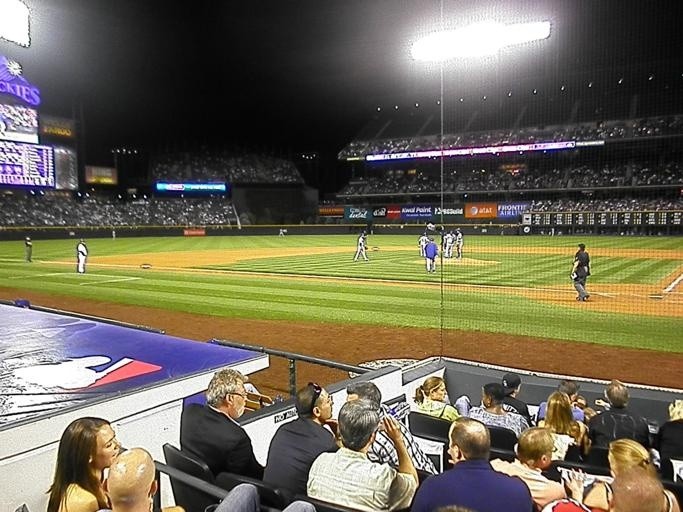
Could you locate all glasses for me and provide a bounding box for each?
[308,382,322,413]
[227,392,246,399]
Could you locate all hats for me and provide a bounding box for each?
[502,372,521,396]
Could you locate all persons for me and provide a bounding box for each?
[279,227,284,236]
[337,114,683,210]
[24,236,33,262]
[77,237,88,274]
[569,243,591,302]
[418,227,463,274]
[353,230,368,261]
[2,148,300,226]
[43,367,683,512]
